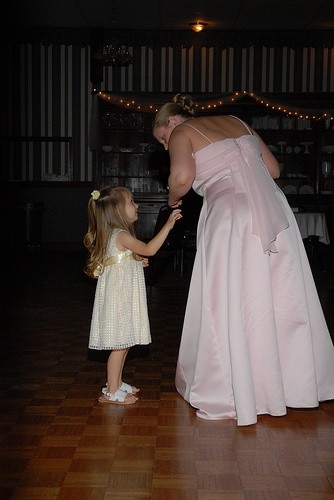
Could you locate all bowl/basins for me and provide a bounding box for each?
[102,145,113,152]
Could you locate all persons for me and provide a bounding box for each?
[153,97,334,426]
[84,185,183,403]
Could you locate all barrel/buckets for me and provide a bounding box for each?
[14,199,44,245]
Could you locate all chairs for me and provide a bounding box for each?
[152,205,188,280]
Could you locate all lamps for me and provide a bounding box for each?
[91,0,135,67]
[189,22,208,33]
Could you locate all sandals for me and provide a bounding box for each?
[98,389,135,405]
[106,382,137,395]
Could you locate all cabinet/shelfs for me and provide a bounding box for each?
[96,92,334,290]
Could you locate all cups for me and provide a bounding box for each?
[102,111,143,129]
